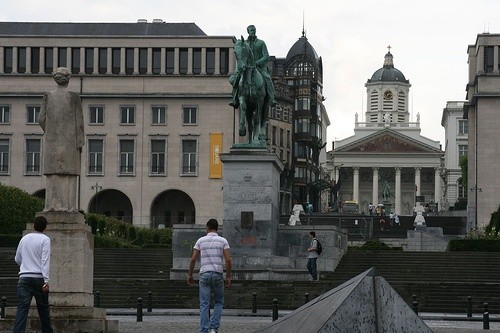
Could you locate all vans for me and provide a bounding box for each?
[341,200,360,215]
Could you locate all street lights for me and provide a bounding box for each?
[470,186,484,231]
[90,182,103,214]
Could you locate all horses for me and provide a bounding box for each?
[381,185,391,201]
[228,33,270,145]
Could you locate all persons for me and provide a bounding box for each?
[369,203,373,215]
[230,25,277,109]
[376,206,386,216]
[187,218,232,333]
[13,216,54,333]
[389,212,400,226]
[379,218,385,232]
[38,66,85,212]
[306,232,319,281]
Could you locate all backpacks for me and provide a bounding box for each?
[312,239,322,255]
